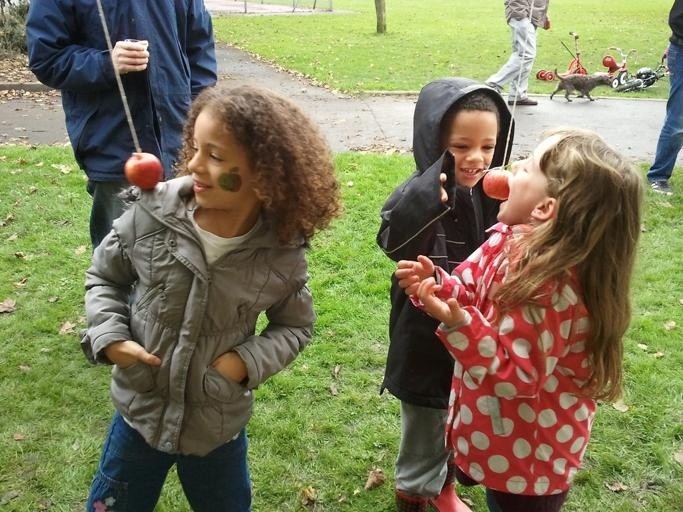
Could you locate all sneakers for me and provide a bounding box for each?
[507,97,538,106]
[651,179,674,197]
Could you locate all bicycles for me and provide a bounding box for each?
[615,63,668,93]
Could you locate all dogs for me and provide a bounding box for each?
[550,68,614,102]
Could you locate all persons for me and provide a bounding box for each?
[26,0,216,251]
[393,128,642,512]
[646,0,682,195]
[485,0,549,105]
[81,85,341,511]
[376,77,513,511]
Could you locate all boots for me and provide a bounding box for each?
[396,460,474,512]
[396,458,474,512]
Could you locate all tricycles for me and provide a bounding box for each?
[594,46,637,88]
[535,31,587,81]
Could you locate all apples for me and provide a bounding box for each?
[125,152,164,191]
[482,168,510,201]
